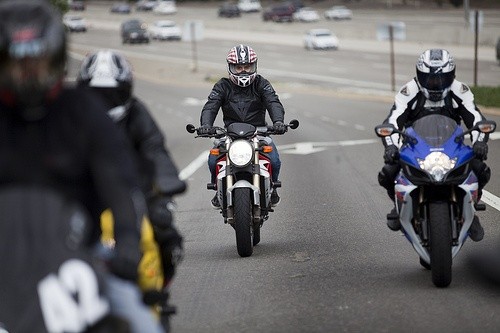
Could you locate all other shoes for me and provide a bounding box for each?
[270,190,280,205]
[470,215,484,242]
[387,207,401,232]
[211,192,221,207]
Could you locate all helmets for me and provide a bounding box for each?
[1,0,70,126]
[77,48,133,123]
[226,44,258,88]
[415,48,456,102]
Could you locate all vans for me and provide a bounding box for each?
[121,20,149,45]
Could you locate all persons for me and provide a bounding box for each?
[0,0,188,330]
[378,48,491,242]
[196,44,287,208]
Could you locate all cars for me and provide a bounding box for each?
[304,30,339,50]
[150,21,182,42]
[66,0,176,16]
[324,6,353,21]
[237,0,263,13]
[291,6,320,22]
[217,1,240,18]
[63,16,87,33]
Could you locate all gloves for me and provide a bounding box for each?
[384,144,400,164]
[197,123,218,135]
[273,121,288,135]
[108,239,146,280]
[473,141,489,160]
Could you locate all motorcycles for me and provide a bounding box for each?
[0,174,188,333]
[374,114,497,288]
[185,119,301,258]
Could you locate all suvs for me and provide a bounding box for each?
[261,3,296,23]
